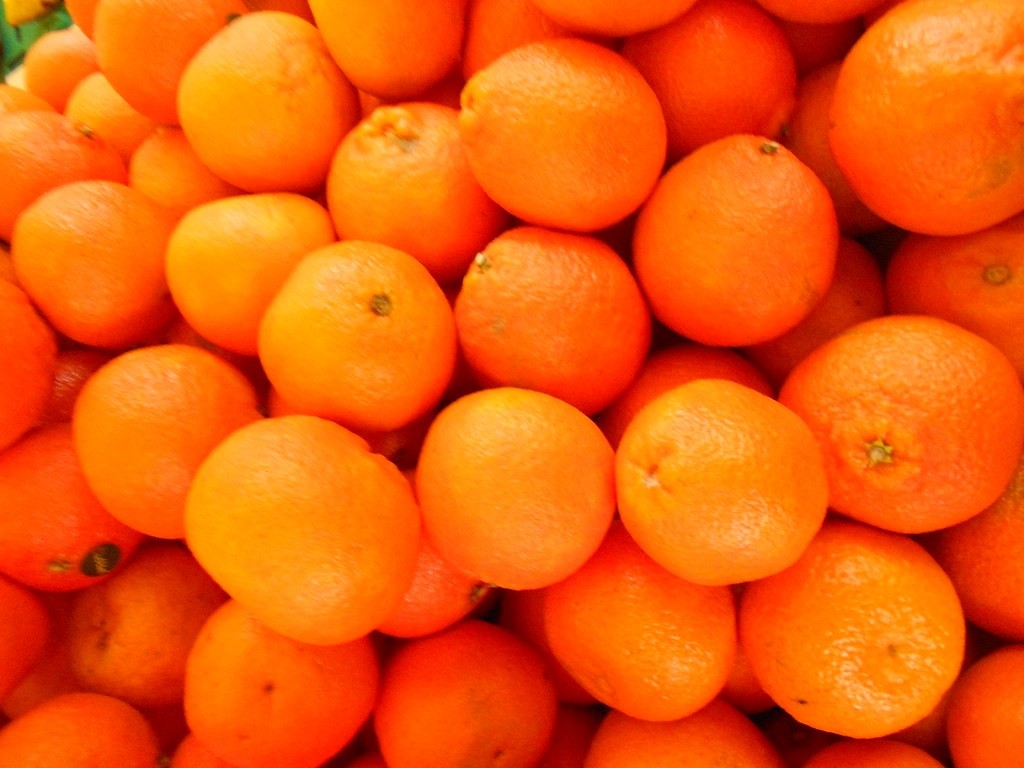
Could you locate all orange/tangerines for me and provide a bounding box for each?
[0,0,1024,768]
